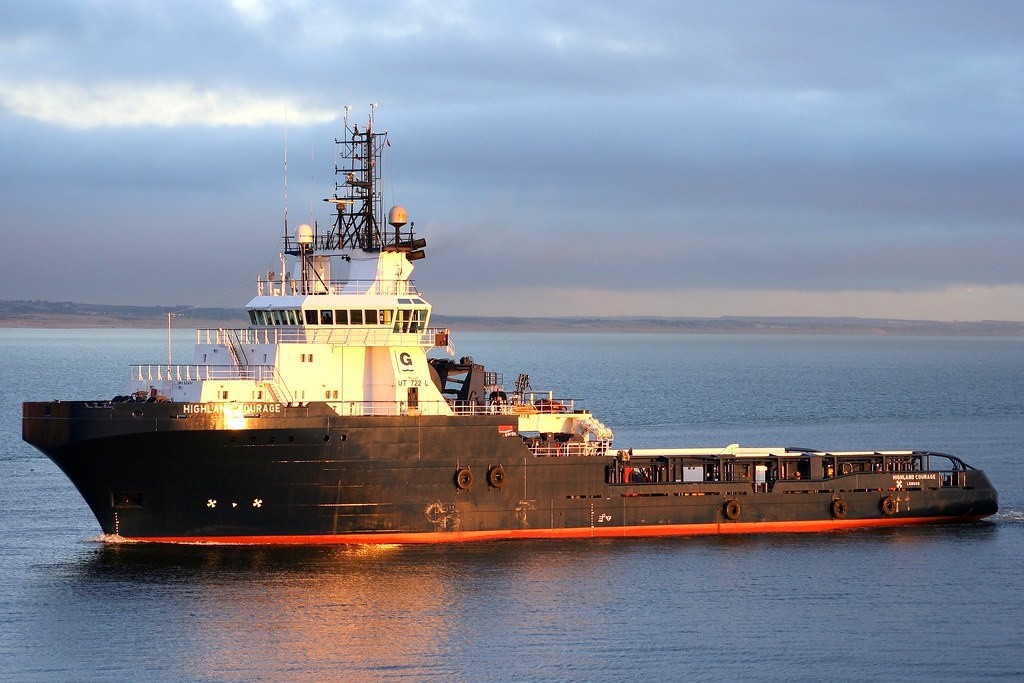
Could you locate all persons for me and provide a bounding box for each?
[792,471,802,494]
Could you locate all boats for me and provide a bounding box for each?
[22,102,997,544]
